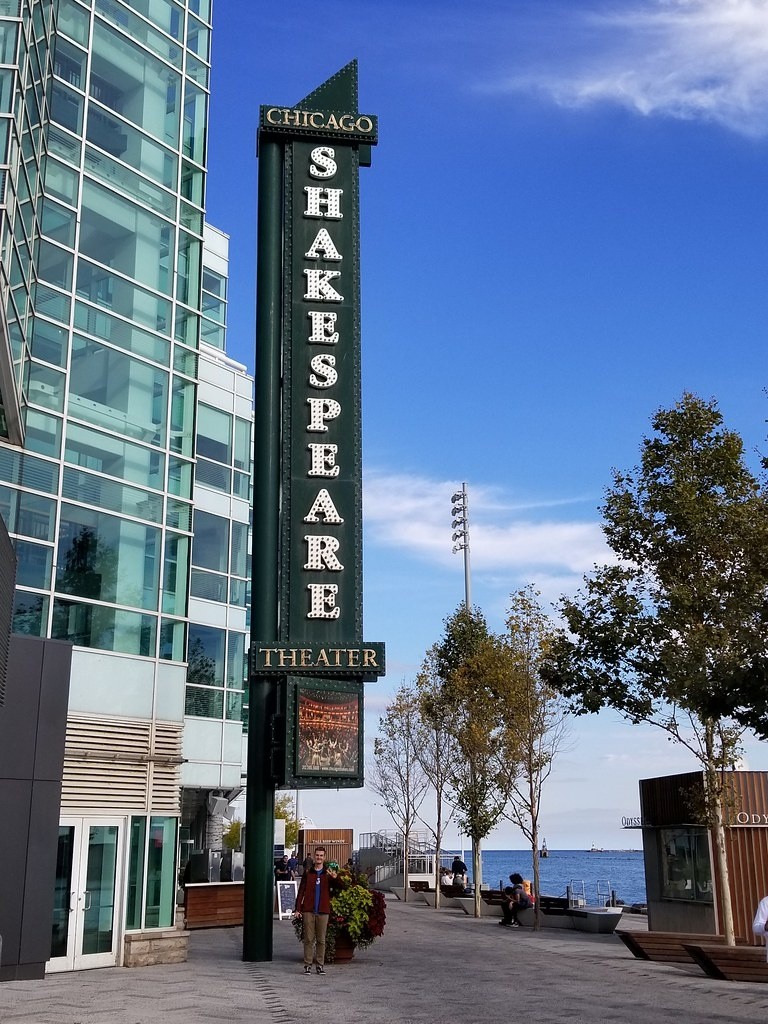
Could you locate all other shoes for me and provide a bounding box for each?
[502,919,505,922]
[509,923,519,928]
[499,922,506,926]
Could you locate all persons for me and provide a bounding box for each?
[295,847,346,975]
[329,858,340,869]
[505,873,533,928]
[297,728,358,769]
[498,886,513,925]
[752,896,768,964]
[288,855,298,880]
[302,853,313,869]
[344,858,353,869]
[440,856,468,886]
[274,855,295,886]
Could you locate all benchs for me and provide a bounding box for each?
[390,885,768,984]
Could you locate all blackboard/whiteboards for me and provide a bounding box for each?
[276,881,298,916]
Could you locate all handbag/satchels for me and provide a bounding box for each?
[464,888,471,894]
[527,893,536,903]
[275,876,283,886]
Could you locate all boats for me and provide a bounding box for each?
[584,848,605,853]
[540,837,548,858]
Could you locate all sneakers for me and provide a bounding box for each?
[315,966,326,975]
[303,966,311,975]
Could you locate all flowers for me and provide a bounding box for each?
[291,861,388,963]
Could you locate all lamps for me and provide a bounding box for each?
[224,805,235,820]
[205,790,229,816]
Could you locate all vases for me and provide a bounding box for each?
[334,930,356,964]
